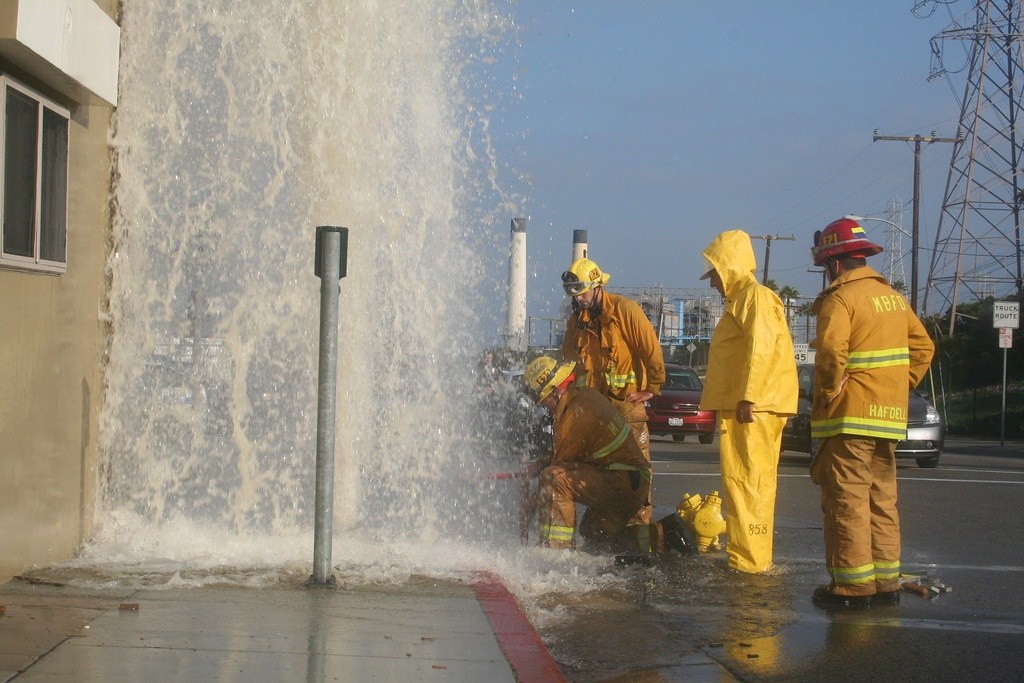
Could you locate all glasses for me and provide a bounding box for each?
[525,384,541,403]
[562,280,590,295]
[811,246,819,259]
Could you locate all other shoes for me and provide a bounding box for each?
[869,591,900,607]
[661,512,698,556]
[811,585,872,613]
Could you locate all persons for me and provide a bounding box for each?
[522,258,697,563]
[809,218,936,606]
[698,230,799,575]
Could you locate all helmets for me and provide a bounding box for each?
[697,256,715,280]
[559,260,606,298]
[523,356,577,405]
[810,218,884,268]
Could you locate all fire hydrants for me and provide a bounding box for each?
[678,488,729,556]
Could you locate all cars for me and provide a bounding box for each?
[781,363,944,468]
[644,361,716,445]
